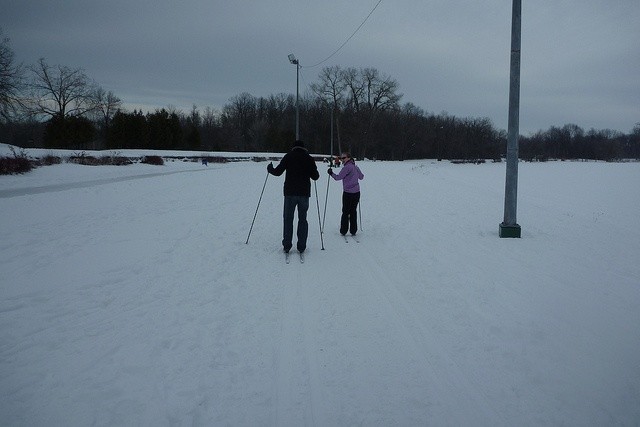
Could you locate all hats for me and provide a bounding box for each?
[293,141,304,146]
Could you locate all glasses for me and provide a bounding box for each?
[340,157,349,160]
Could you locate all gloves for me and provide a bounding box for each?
[328,168,333,174]
[267,163,274,173]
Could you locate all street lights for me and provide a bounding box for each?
[288,54,299,140]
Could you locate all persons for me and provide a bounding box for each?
[323,157,328,162]
[329,155,333,166]
[201,158,207,165]
[268,141,319,254]
[327,151,364,236]
[335,157,341,167]
